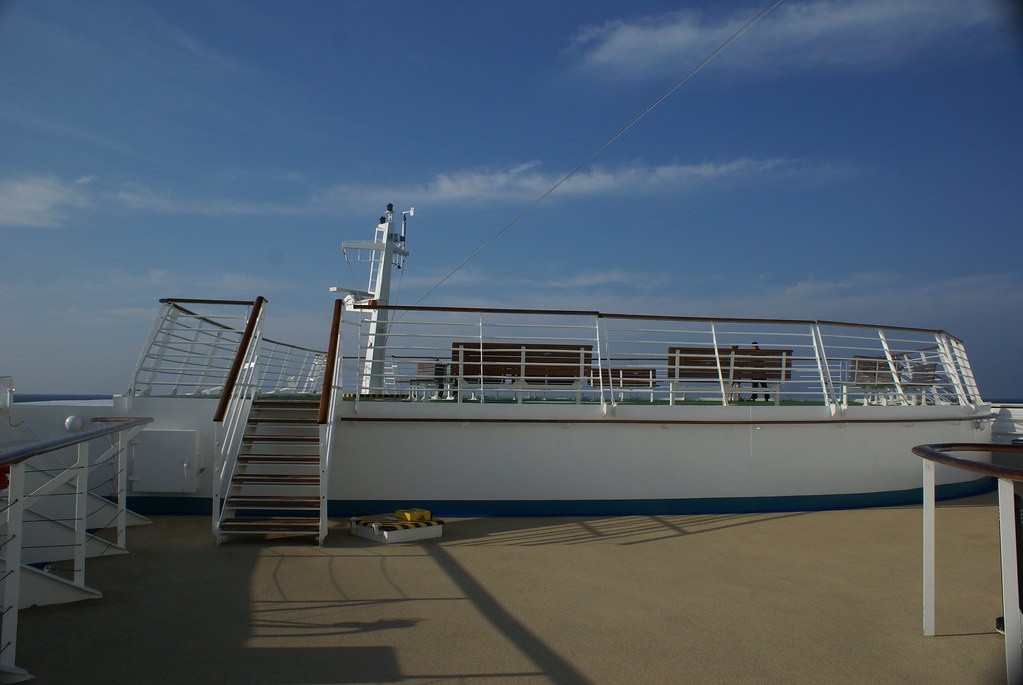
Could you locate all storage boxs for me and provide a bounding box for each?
[396,508,430,521]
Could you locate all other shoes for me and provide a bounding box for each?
[736,397,743,401]
[765,399,768,401]
[728,397,733,401]
[746,398,755,401]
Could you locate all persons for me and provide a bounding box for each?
[746,342,770,402]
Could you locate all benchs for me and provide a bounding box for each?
[447,341,594,400]
[666,346,796,406]
[590,366,660,401]
[396,358,447,401]
[826,355,904,406]
[901,360,940,403]
